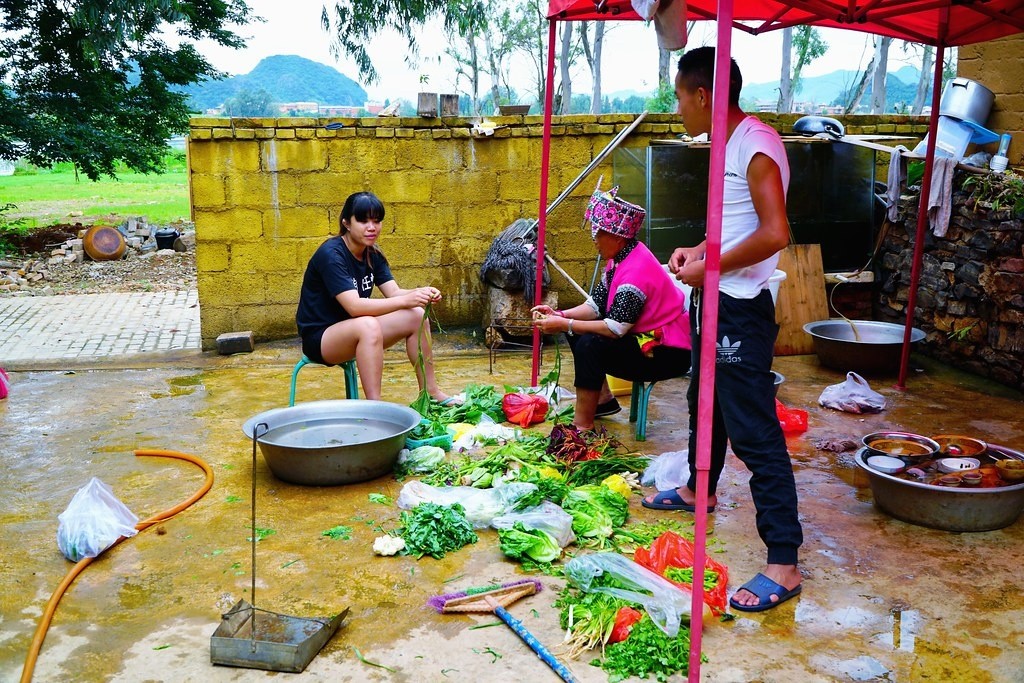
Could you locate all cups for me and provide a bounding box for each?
[991,155,1009,170]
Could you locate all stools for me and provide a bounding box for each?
[630,370,692,441]
[290,353,359,407]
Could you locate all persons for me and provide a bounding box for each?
[530,189,692,438]
[642,47,804,633]
[296,192,464,407]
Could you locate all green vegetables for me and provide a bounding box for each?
[432,383,576,423]
[551,571,709,682]
[391,424,654,511]
[386,501,479,559]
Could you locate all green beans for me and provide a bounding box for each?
[662,565,720,589]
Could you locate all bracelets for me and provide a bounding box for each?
[568,318,574,336]
[556,310,564,317]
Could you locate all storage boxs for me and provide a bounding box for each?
[915,117,976,160]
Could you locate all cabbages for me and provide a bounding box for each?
[498,521,562,563]
[562,483,629,550]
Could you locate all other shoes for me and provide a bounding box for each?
[594,398,622,416]
[589,428,598,437]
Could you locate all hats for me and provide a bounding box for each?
[581,174,646,241]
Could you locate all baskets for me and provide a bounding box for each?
[406,419,456,452]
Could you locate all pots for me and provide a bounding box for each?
[83,224,126,262]
[155,226,180,249]
[794,117,845,138]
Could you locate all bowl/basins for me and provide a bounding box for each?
[854,432,1024,531]
[242,398,422,487]
[801,319,926,375]
[499,104,531,116]
[770,370,786,397]
[662,265,787,311]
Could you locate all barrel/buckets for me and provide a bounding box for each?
[939,76,996,125]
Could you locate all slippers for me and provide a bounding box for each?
[729,572,802,612]
[439,398,464,407]
[642,487,715,513]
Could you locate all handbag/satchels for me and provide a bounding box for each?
[635,530,729,618]
[775,398,809,433]
[565,552,712,637]
[819,372,886,414]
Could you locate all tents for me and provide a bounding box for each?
[532,0,1024,388]
